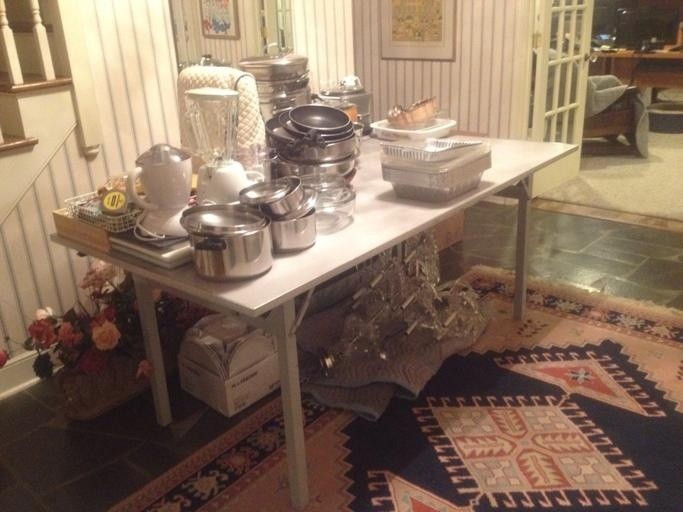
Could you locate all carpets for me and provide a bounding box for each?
[104,263,683,512]
[536,86,683,221]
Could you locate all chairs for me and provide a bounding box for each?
[529,47,649,157]
[178,65,265,156]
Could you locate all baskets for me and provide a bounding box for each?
[64,191,142,234]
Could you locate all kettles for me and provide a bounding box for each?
[127,142,192,242]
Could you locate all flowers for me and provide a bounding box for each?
[0,251,183,380]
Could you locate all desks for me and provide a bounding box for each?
[590,50,683,74]
[50,133,578,512]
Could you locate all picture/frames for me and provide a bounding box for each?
[199,0,241,40]
[379,0,457,62]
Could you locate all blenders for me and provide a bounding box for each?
[183,88,249,209]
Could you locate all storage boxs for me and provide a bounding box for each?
[297,210,465,319]
[178,311,282,418]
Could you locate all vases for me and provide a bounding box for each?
[54,363,148,420]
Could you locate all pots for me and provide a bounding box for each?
[242,42,372,133]
[179,106,360,283]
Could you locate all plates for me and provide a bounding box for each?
[180,314,269,376]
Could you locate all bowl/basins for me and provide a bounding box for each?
[591,39,616,48]
